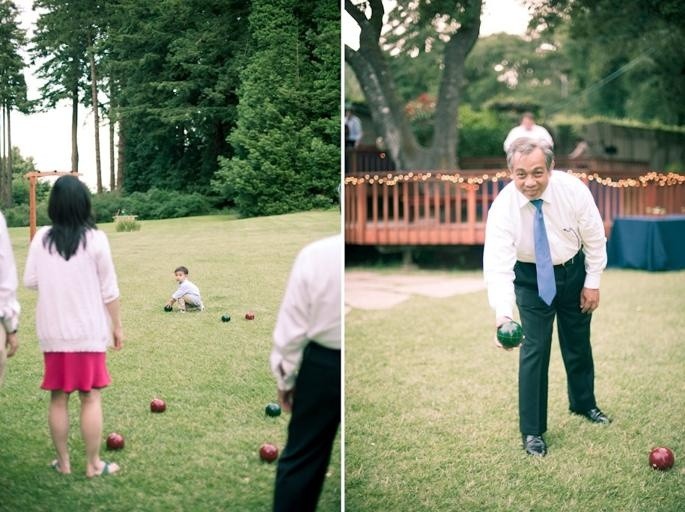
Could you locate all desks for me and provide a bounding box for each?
[606,215,685,270]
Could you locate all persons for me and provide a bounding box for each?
[163,265,205,313]
[502,112,555,154]
[570,137,594,161]
[270,184,340,510]
[0,204,22,385]
[345,108,363,147]
[165,305,171,312]
[481,138,613,459]
[25,174,124,476]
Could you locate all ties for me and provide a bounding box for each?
[529,199,557,306]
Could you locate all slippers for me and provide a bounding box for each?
[51,459,63,473]
[100,461,121,475]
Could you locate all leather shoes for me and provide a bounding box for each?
[585,407,608,424]
[521,434,546,457]
